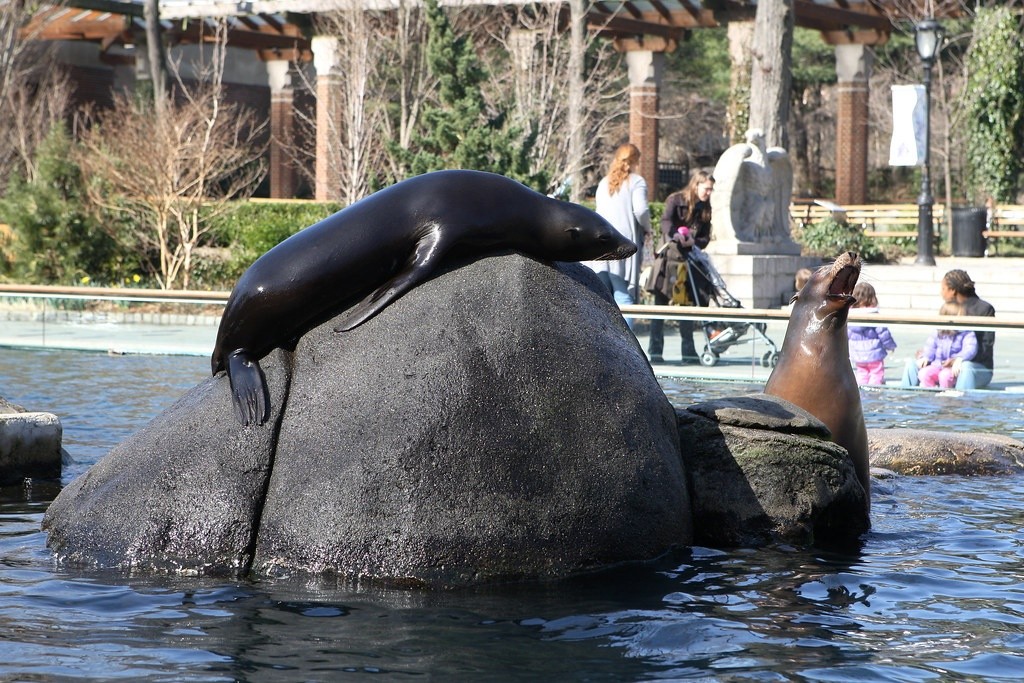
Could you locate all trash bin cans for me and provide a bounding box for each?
[950,206,988,257]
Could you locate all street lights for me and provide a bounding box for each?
[911,18,948,270]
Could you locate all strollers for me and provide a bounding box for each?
[657,238,781,369]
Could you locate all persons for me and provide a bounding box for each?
[846,283,897,384]
[917,303,978,387]
[795,267,812,291]
[647,170,716,366]
[595,144,651,331]
[901,269,996,389]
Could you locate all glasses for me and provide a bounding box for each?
[699,184,714,192]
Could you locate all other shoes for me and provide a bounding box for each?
[683,351,699,363]
[649,355,665,364]
